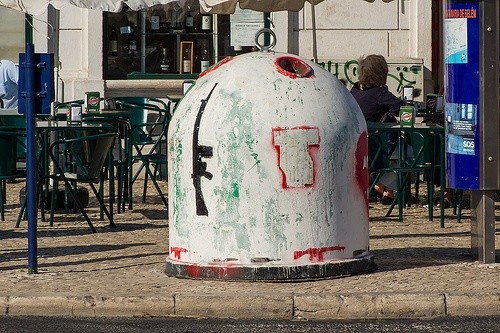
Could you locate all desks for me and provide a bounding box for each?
[54,108,134,209]
[34,120,114,221]
[367,122,447,222]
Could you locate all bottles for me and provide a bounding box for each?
[160,48,170,73]
[185,9,194,33]
[128,28,136,51]
[200,44,210,72]
[108,23,119,56]
[201,14,212,33]
[182,44,190,74]
[150,5,161,33]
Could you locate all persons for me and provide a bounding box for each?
[0,60,19,183]
[350,54,404,205]
[145,35,173,73]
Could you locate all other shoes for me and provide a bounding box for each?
[436,197,450,207]
[406,191,420,204]
[372,182,396,205]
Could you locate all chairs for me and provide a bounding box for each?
[0,95,426,231]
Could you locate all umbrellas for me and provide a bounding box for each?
[0,0,178,187]
[200,0,404,65]
